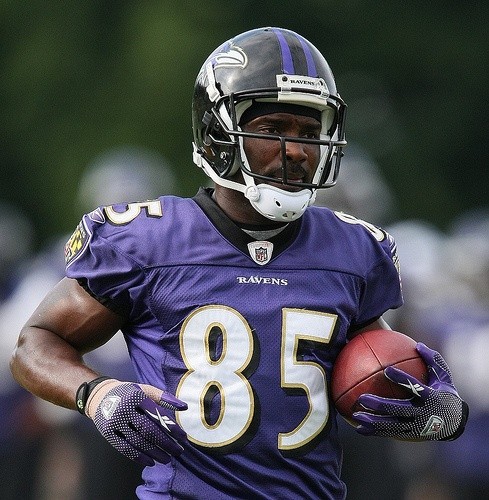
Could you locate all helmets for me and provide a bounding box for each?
[191,26,346,223]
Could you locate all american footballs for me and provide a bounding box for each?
[330,328,436,420]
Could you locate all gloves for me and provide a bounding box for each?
[83,378,189,467]
[352,342,469,442]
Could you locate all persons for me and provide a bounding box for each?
[9,27,469,500]
